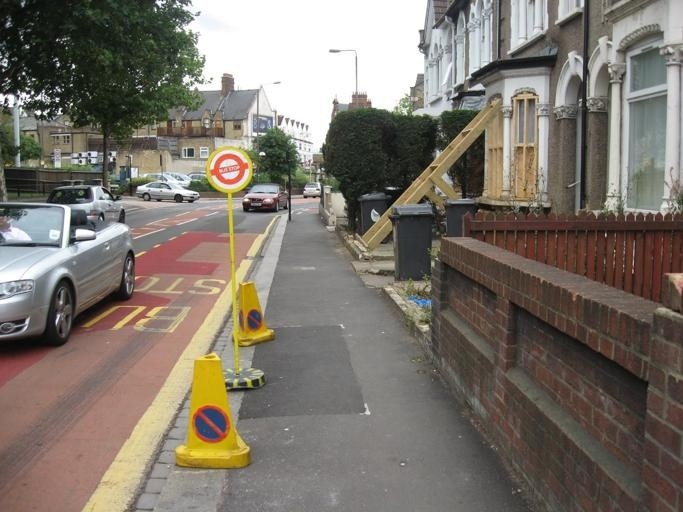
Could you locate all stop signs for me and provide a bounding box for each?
[206,146,254,194]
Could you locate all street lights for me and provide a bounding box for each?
[128,154,133,196]
[329,49,359,105]
[257,81,282,183]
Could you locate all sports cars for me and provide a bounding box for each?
[0,201,136,346]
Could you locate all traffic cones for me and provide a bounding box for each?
[232,282,275,347]
[175,353,252,469]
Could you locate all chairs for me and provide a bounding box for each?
[68,211,97,241]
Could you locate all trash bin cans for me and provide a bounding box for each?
[388,199,475,280]
[358,193,392,243]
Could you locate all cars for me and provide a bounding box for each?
[241,183,288,212]
[303,182,322,198]
[143,171,207,189]
[47,184,126,224]
[136,181,200,202]
